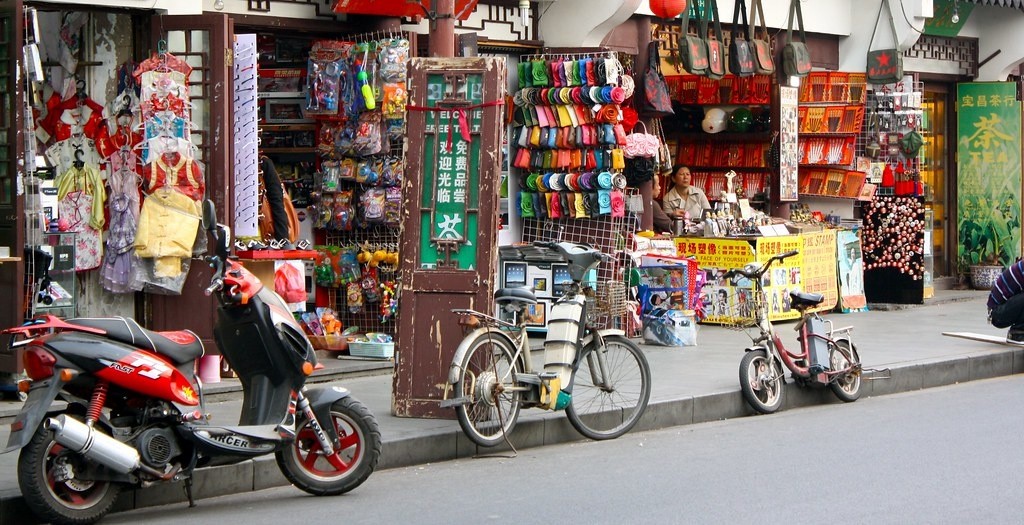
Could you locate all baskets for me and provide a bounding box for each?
[562,280,628,317]
[714,290,764,331]
[346,341,394,357]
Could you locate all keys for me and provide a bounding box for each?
[987,316,992,325]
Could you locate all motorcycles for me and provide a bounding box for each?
[0,198,382,525]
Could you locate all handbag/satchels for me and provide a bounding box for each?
[622,120,659,158]
[783,0,812,76]
[625,188,644,213]
[635,42,674,117]
[651,119,673,176]
[866,0,903,84]
[678,0,775,80]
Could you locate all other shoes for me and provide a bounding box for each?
[1006,330,1024,345]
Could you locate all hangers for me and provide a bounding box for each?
[68,40,199,189]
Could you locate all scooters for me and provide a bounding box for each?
[438,220,651,461]
[723,249,863,414]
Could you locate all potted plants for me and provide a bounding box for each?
[969,227,1016,291]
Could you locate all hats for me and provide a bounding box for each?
[901,131,923,158]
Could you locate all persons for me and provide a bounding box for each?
[987,259,1024,346]
[773,293,779,313]
[725,170,737,193]
[703,208,761,237]
[782,289,791,312]
[849,247,863,295]
[663,163,712,222]
[306,63,322,110]
[707,266,754,316]
[653,173,686,234]
[258,154,299,250]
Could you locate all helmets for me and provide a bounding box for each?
[702,107,771,135]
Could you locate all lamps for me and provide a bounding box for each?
[519,0,530,27]
